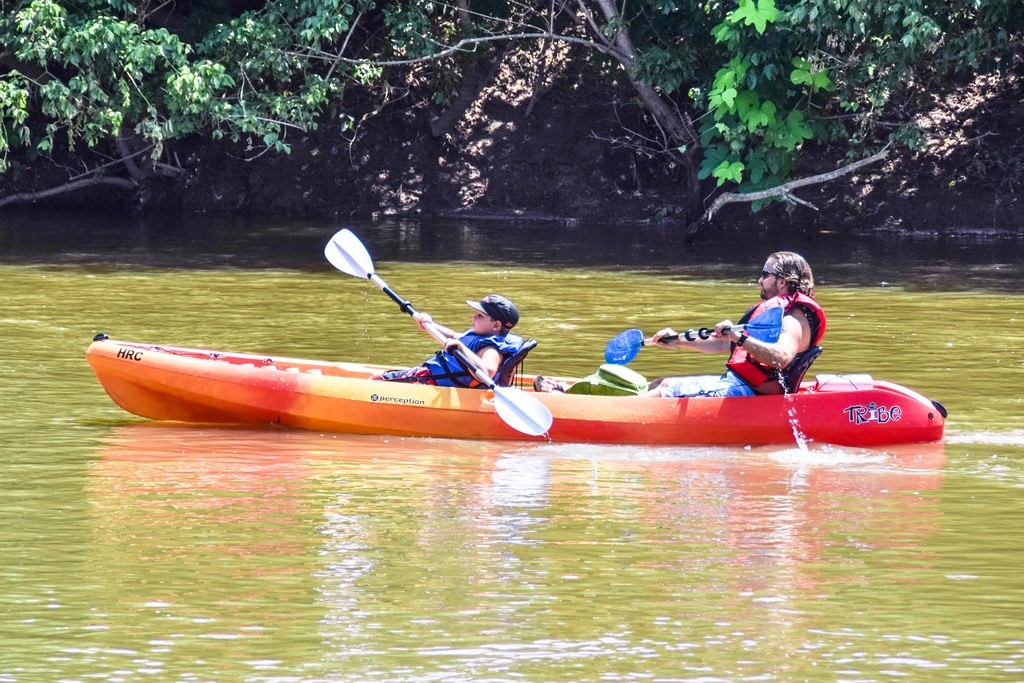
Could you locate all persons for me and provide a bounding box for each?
[533,251,828,399]
[370,294,519,388]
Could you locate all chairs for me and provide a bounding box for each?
[786,347,823,393]
[492,339,537,389]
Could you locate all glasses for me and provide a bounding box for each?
[761,271,778,279]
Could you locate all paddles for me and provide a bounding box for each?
[604,305,786,364]
[321,227,555,437]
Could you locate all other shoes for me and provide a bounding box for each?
[534,376,565,394]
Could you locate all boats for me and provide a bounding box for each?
[91,422,943,503]
[85,333,947,447]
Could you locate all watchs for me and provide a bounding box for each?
[737,333,747,346]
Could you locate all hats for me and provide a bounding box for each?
[466,294,519,328]
